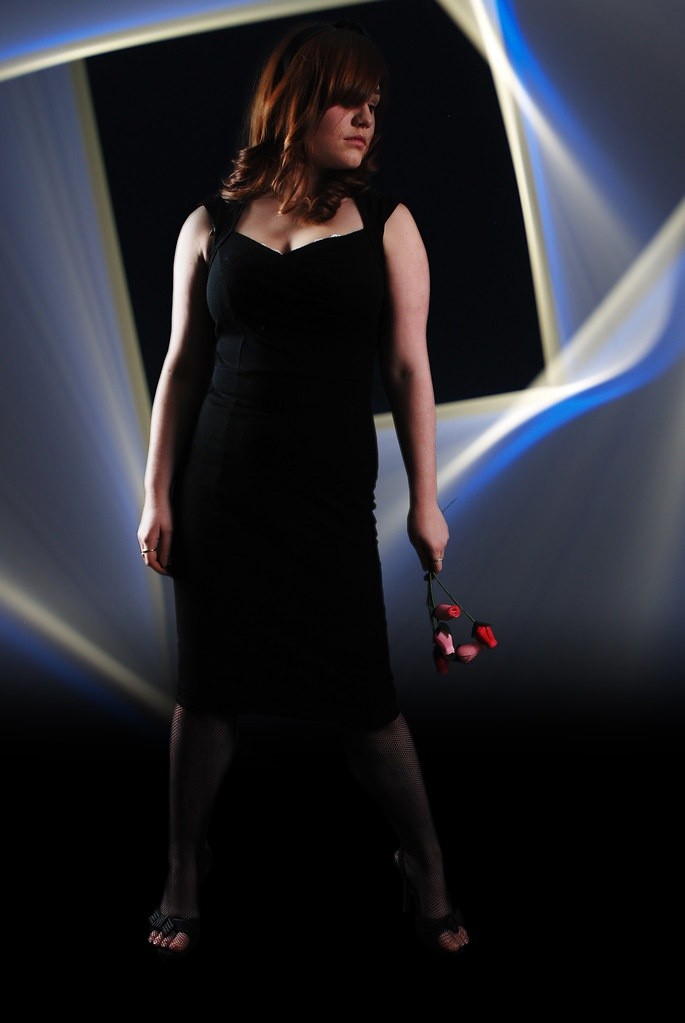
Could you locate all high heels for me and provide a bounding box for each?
[145,896,201,959]
[393,846,466,956]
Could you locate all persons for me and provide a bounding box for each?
[137,26,472,955]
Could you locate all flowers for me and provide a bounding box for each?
[423,559,501,675]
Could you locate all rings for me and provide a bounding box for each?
[432,556,446,562]
[140,547,158,558]
